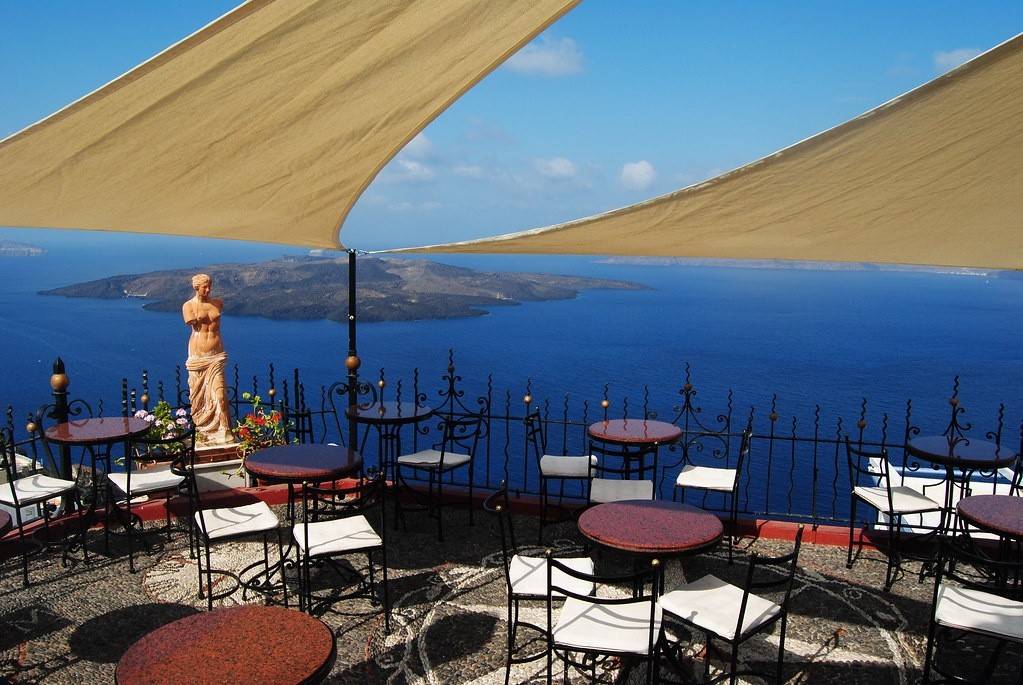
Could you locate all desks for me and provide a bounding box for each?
[578,499,724,685]
[241,443,362,616]
[43,416,152,567]
[932,494,1023,685]
[114,606,338,685]
[895,435,1016,581]
[582,417,683,559]
[345,401,431,517]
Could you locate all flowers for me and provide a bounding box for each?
[222,393,299,479]
[136,402,191,457]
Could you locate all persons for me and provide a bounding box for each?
[182,274,235,444]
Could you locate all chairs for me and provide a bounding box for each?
[844,435,1023,685]
[0,408,804,685]
